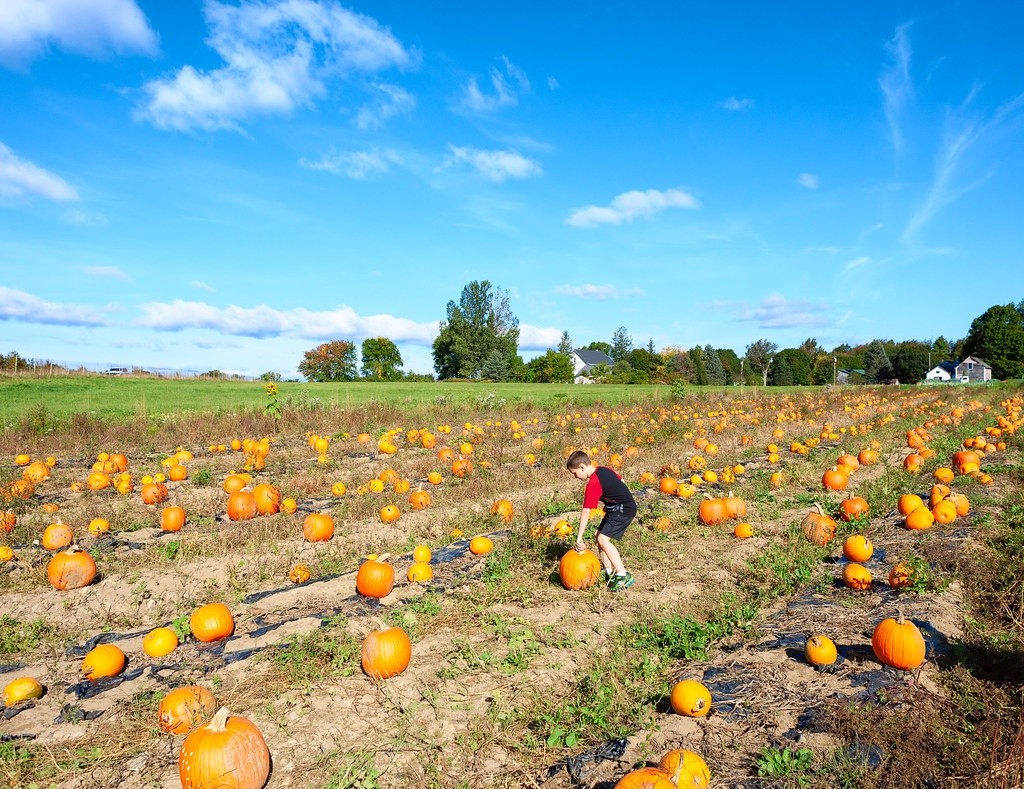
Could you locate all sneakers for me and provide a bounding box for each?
[605,572,635,592]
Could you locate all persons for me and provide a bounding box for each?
[565,449,638,593]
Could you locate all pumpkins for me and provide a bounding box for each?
[1,392,1024,789]
